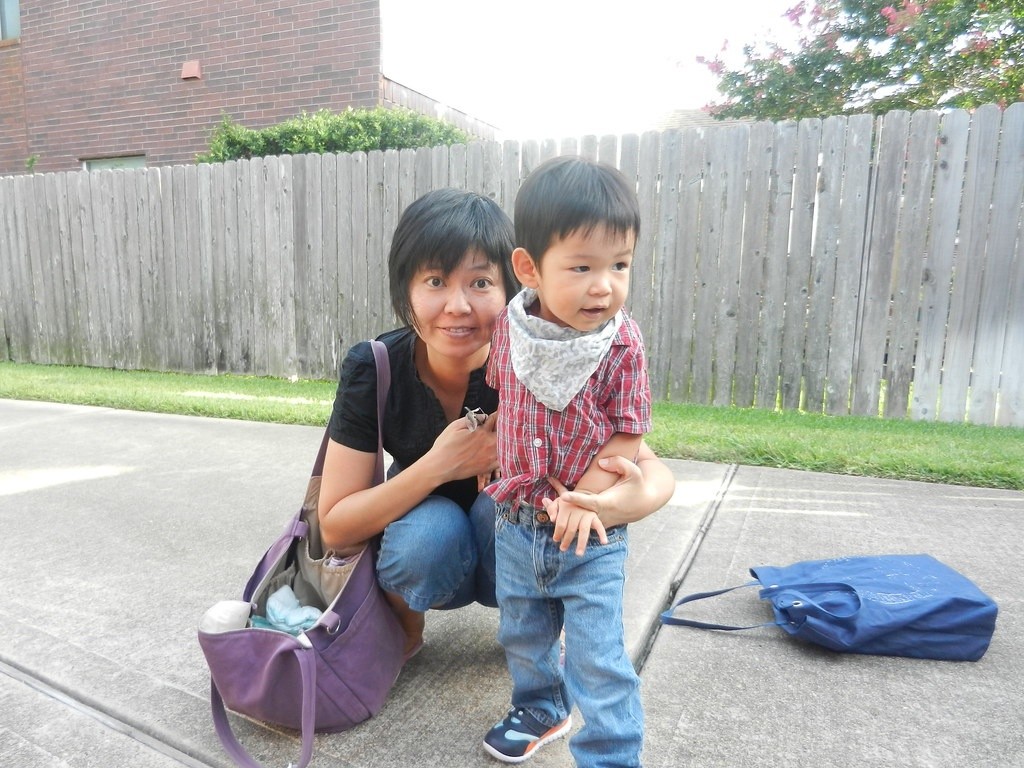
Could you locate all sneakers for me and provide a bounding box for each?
[483,707,571,763]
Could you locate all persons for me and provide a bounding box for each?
[482,155,652,768]
[317,189,675,664]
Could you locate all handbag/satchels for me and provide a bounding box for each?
[661,552,998,663]
[198,338,413,768]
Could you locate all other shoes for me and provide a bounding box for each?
[407,636,424,660]
